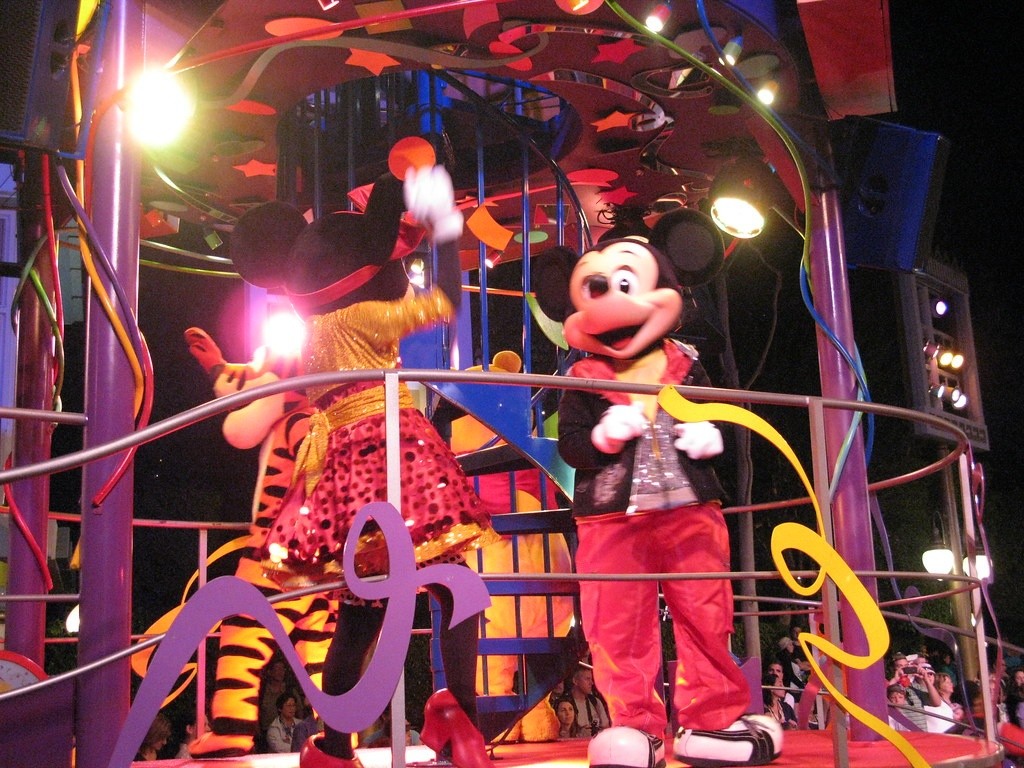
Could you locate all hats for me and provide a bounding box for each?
[890,653,906,662]
[886,684,906,701]
[921,663,936,675]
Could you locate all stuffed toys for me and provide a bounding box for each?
[184,326,359,758]
[229,168,501,768]
[448,350,577,741]
[531,207,784,768]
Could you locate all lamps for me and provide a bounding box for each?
[646,0,674,32]
[923,340,965,370]
[928,383,968,409]
[757,67,782,104]
[708,156,777,238]
[718,24,744,68]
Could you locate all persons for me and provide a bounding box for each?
[764,616,1024,768]
[256,657,325,755]
[175,708,213,758]
[545,647,612,739]
[133,714,172,761]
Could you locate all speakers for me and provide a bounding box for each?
[0,0,80,155]
[841,115,953,276]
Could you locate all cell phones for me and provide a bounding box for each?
[902,667,918,674]
